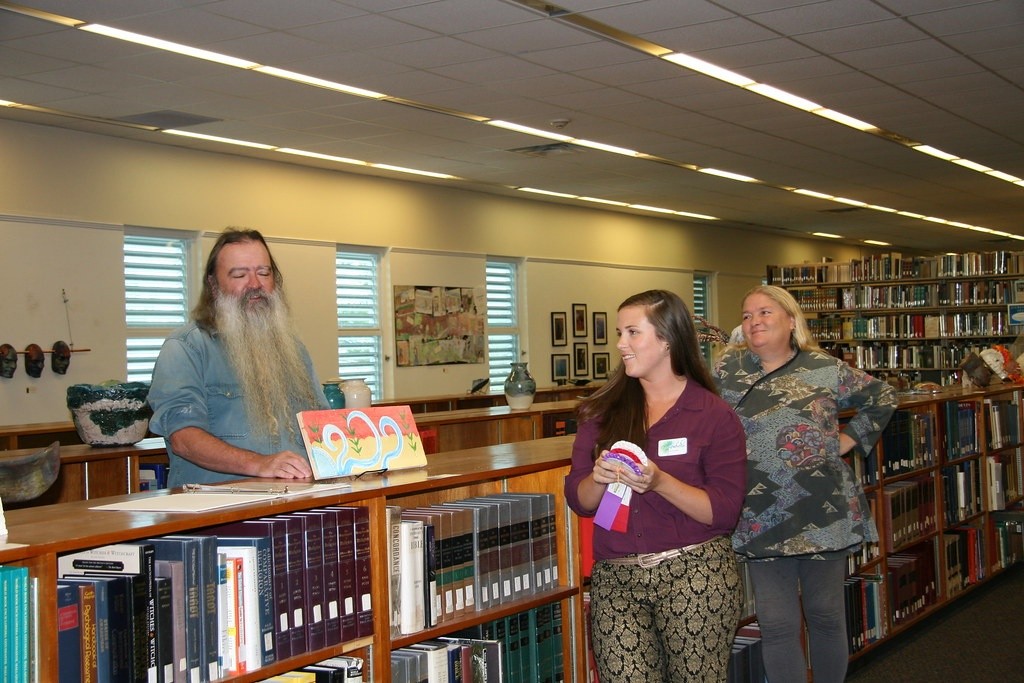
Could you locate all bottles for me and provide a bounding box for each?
[504,362,536,410]
[320,377,372,409]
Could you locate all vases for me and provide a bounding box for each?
[504,362,537,410]
[321,378,372,407]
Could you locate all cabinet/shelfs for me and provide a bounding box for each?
[774,273,1024,373]
[0,384,1024,683]
[0,399,578,509]
[0,384,600,451]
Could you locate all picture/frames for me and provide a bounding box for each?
[552,354,570,381]
[574,342,589,376]
[572,303,588,337]
[592,352,610,380]
[551,312,568,347]
[592,311,608,345]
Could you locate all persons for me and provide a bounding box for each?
[566,289,747,683]
[716,285,898,683]
[147,229,335,488]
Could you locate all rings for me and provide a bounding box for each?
[641,475,646,485]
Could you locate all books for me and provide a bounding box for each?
[836,393,1024,658]
[0,492,562,683]
[724,559,768,683]
[766,251,1024,386]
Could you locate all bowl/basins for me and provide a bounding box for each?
[65,381,152,448]
